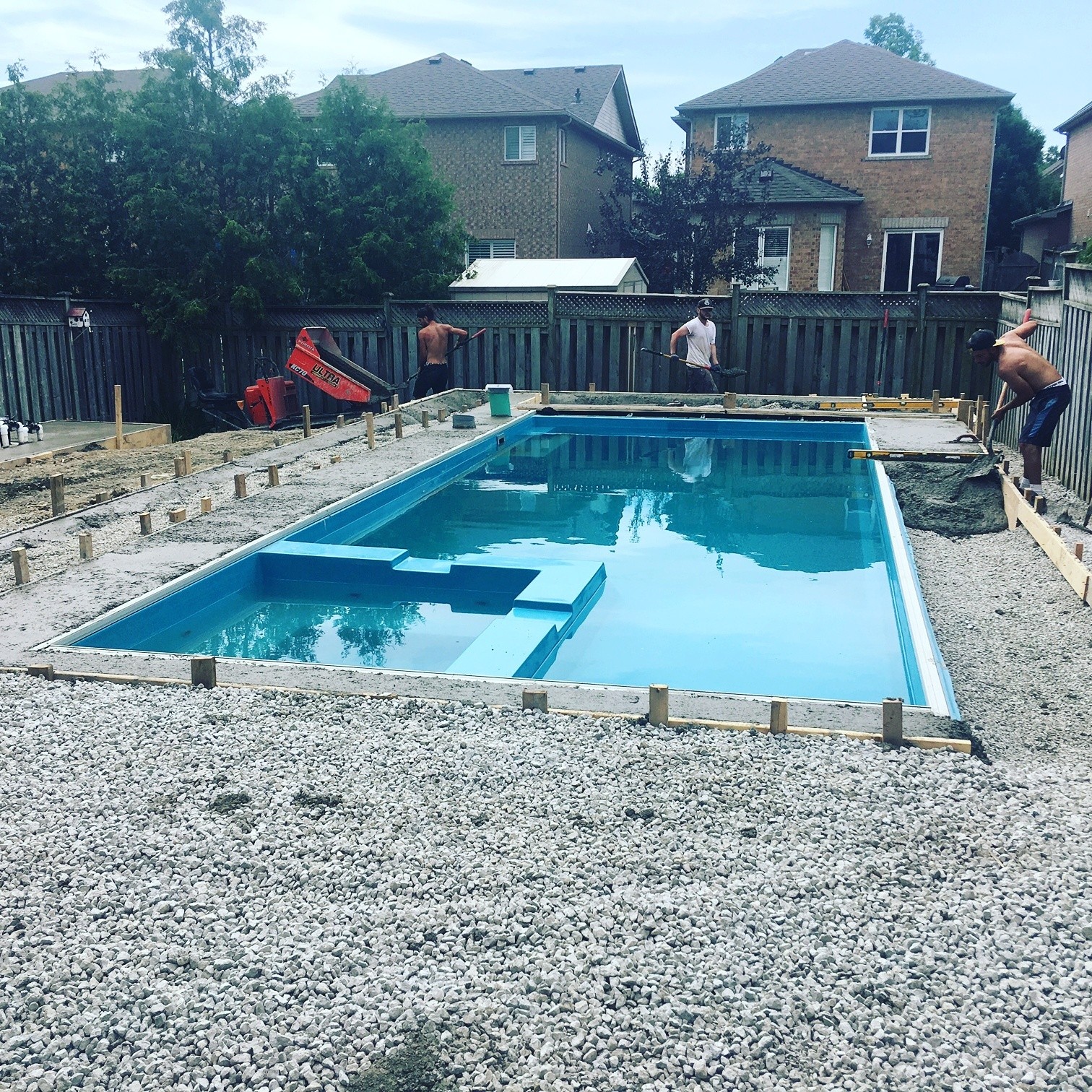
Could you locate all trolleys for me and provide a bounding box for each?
[243,326,393,426]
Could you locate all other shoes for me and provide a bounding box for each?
[1018,487,1049,513]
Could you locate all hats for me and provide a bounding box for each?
[697,299,715,309]
[966,329,1006,353]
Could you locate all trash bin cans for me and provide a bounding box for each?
[936,276,970,292]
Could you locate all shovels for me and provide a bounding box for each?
[964,309,1032,478]
[386,328,488,390]
[641,347,748,377]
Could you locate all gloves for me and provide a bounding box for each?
[713,364,722,374]
[671,354,680,361]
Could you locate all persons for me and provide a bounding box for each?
[411,306,468,400]
[965,321,1072,502]
[670,298,722,394]
